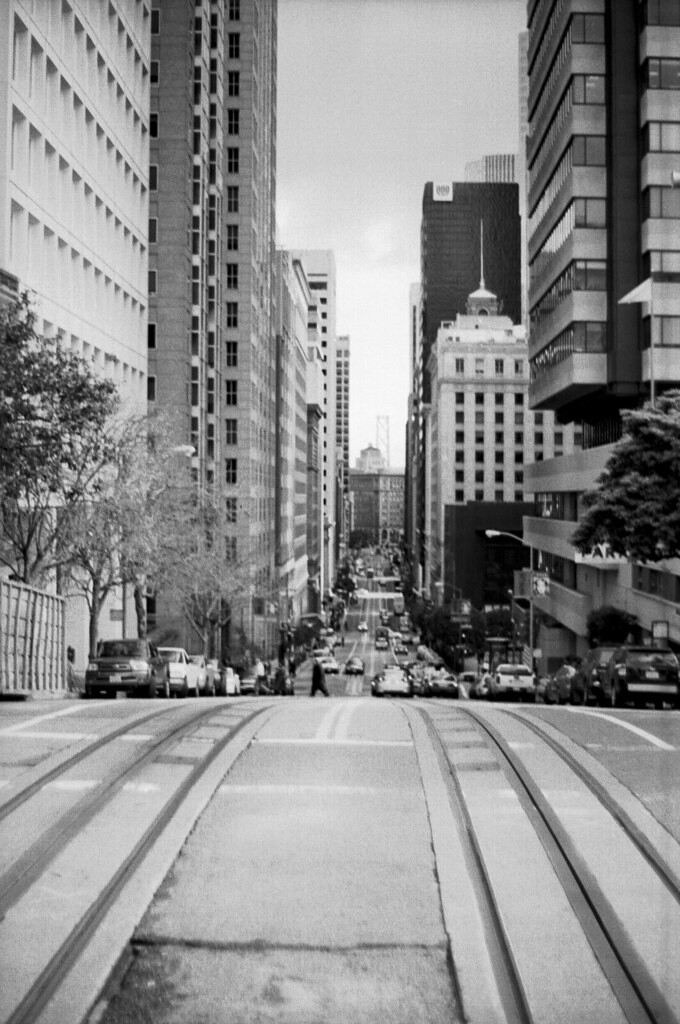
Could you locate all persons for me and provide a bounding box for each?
[252,658,271,696]
[309,660,330,697]
[274,663,287,696]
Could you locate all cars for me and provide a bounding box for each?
[83,639,295,699]
[310,607,680,709]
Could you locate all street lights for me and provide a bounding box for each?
[484,527,534,675]
[116,443,196,640]
